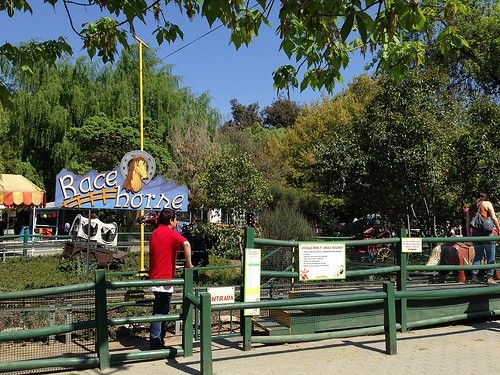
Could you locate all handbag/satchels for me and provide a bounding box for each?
[469,202,494,233]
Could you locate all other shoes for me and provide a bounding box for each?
[471,278,485,284]
[484,279,498,286]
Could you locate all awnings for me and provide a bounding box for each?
[0,173,46,209]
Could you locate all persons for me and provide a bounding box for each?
[466,191,500,285]
[148,208,195,350]
